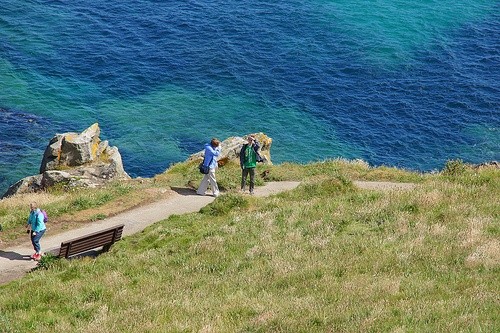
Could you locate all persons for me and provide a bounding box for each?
[197,138,221,197]
[24,202,48,261]
[239,135,263,193]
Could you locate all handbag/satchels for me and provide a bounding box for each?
[199,165,210,174]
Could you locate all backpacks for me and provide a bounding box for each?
[36,209,47,223]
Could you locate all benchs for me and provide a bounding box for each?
[44,223,125,260]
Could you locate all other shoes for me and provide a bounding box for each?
[31,253,41,261]
